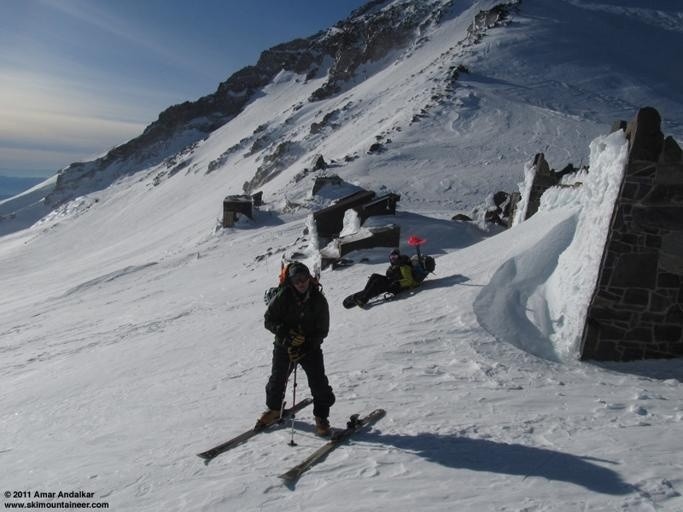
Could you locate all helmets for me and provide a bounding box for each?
[387,250,398,263]
[289,262,309,277]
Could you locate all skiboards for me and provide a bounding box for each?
[197,386,386,490]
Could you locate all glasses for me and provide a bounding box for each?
[291,275,308,283]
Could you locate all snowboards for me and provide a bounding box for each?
[342,286,395,309]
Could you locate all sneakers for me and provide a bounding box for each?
[260,410,280,424]
[315,417,329,435]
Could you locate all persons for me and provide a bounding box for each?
[353,248,415,308]
[262,259,336,437]
